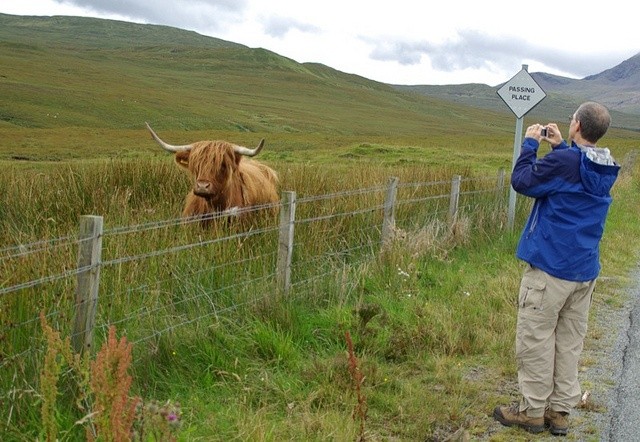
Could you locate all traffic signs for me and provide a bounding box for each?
[496,68,547,120]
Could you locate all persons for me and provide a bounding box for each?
[492,101,622,436]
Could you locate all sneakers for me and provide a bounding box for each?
[493,405,544,435]
[544,406,569,436]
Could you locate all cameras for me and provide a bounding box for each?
[540,128,549,138]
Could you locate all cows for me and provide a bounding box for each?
[143,120,282,249]
